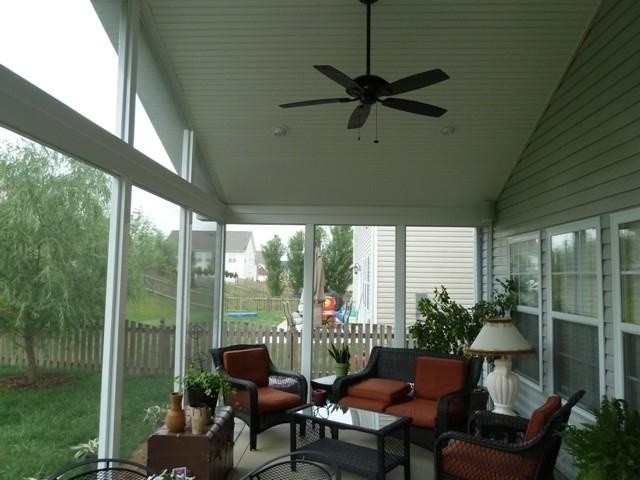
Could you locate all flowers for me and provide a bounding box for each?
[147,466,196,480]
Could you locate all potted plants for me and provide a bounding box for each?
[328,343,350,376]
[166,356,236,417]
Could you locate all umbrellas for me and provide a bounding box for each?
[313,240,327,303]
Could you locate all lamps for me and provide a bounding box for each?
[469,317,534,416]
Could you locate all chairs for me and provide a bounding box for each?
[47,457,147,480]
[238,450,341,480]
[433,388,586,480]
[206,344,308,450]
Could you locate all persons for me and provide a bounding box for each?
[325,282,348,323]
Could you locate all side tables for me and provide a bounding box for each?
[147,405,234,480]
[309,374,351,427]
[472,408,530,441]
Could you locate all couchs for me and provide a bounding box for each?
[329,345,484,453]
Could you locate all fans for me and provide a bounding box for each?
[278,0,450,144]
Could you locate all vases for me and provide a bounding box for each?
[166,392,186,432]
